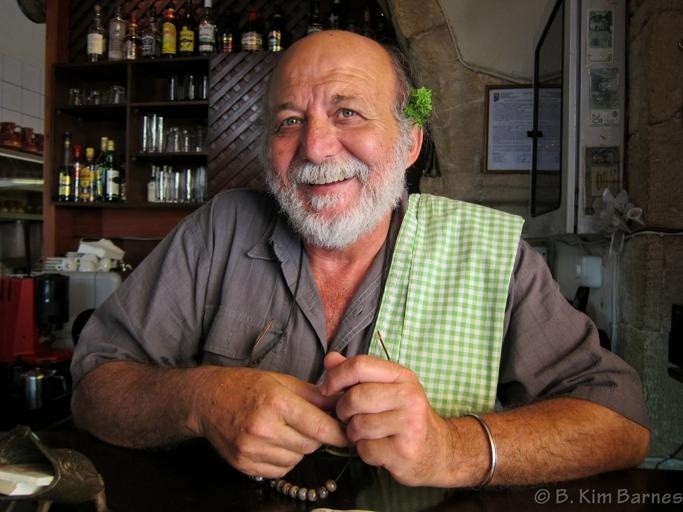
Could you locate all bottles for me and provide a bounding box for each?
[86,0,219,62]
[57,132,126,201]
[240,12,264,52]
[168,74,209,100]
[21,369,46,411]
[307,9,323,33]
[141,112,206,152]
[69,86,126,103]
[268,15,288,55]
[359,9,373,39]
[147,163,207,204]
[222,29,235,54]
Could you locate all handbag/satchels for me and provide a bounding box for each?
[0,424,107,512]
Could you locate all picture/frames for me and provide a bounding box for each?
[575,1,629,236]
[539,88,563,172]
[483,85,537,175]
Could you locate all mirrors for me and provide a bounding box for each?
[525,1,564,217]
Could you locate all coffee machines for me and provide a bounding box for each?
[1,274,69,367]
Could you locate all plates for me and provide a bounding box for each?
[44,257,65,271]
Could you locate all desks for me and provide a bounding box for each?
[0,434,683,512]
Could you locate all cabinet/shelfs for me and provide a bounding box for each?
[43,0,393,270]
[0,146,43,278]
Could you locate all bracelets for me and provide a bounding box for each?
[464,413,497,492]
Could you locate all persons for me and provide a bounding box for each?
[67,28,652,491]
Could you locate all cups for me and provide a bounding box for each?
[56,253,118,272]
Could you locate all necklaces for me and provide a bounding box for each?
[250,209,397,501]
